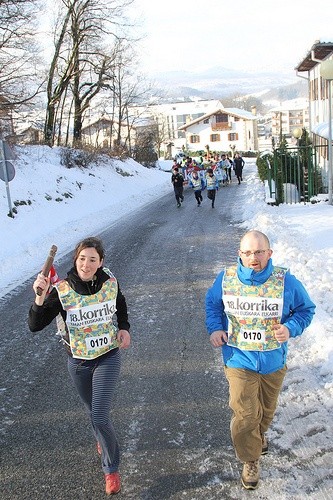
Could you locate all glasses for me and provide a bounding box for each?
[240,249,269,256]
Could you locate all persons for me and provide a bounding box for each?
[28,237,131,494]
[204,231,316,490]
[171,151,245,208]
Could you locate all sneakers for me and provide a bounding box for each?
[261,434,268,454]
[104,471,120,495]
[97,441,101,454]
[241,459,259,490]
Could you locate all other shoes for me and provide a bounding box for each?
[176,203,180,207]
[180,198,183,202]
